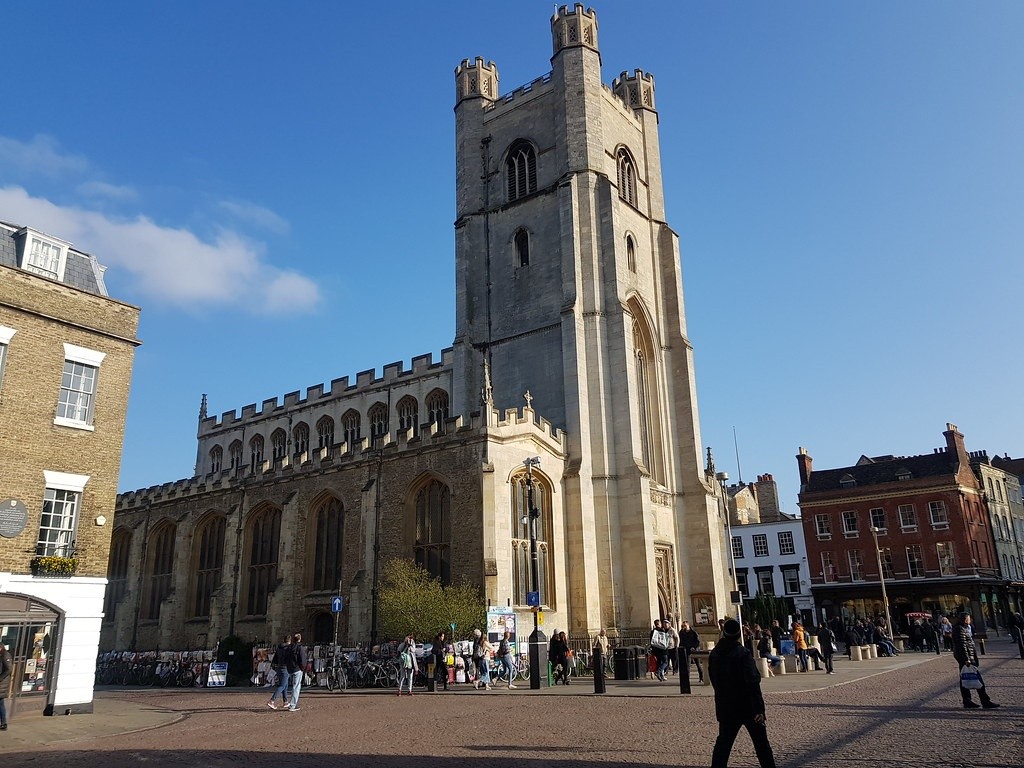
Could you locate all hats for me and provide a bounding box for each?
[723,620,741,640]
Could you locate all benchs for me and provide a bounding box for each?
[851,645,872,661]
[868,643,881,658]
[786,654,814,673]
[755,656,787,678]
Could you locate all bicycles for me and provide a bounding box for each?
[355,653,450,688]
[567,642,621,675]
[326,656,347,692]
[497,653,530,682]
[96,658,208,688]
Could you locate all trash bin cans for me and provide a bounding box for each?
[613,647,636,681]
[630,645,646,680]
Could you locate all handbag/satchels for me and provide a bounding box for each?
[830,641,838,653]
[561,655,575,669]
[650,629,670,651]
[960,664,983,690]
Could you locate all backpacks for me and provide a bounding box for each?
[473,644,486,660]
[804,631,812,647]
[498,646,504,658]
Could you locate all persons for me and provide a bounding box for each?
[1007,610,1020,643]
[396,623,573,696]
[592,626,611,679]
[266,634,294,711]
[951,610,1002,710]
[1015,612,1024,641]
[709,617,778,768]
[284,633,307,711]
[718,611,978,674]
[648,618,705,683]
[0,642,13,729]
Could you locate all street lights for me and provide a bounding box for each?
[870,526,893,639]
[715,471,745,647]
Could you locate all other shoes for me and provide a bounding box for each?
[288,705,300,712]
[267,699,277,710]
[983,701,1000,708]
[964,700,980,708]
[881,644,954,657]
[767,656,837,677]
[395,669,704,697]
[0,724,8,731]
[283,701,290,707]
